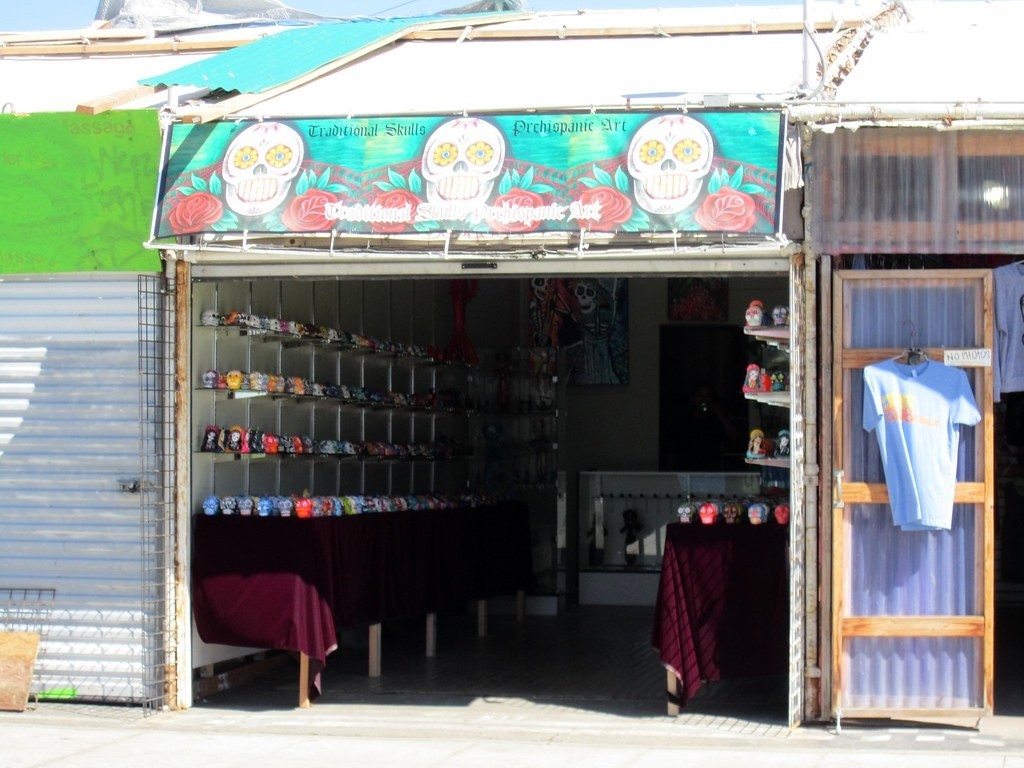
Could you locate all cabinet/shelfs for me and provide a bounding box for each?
[197,322,479,469]
[742,326,792,470]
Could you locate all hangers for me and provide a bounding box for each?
[863,319,966,390]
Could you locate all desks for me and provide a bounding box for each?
[191,499,535,707]
[665,494,788,715]
